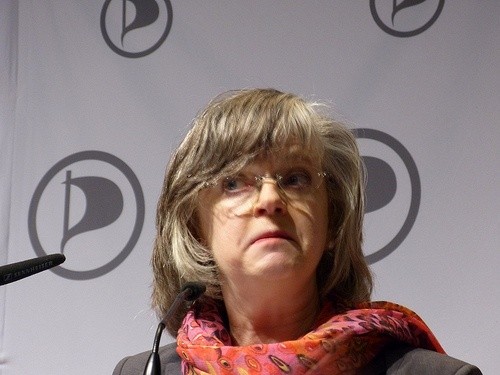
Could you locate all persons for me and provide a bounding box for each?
[112,87,484,374]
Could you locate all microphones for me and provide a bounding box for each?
[143,281,206,375]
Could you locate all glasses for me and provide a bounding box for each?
[204,162,328,195]
